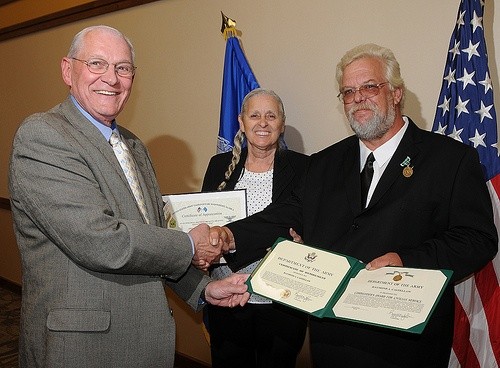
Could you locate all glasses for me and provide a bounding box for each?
[72,57,138,77]
[337,81,389,104]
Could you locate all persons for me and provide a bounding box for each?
[8,24,252,368]
[192,44,498,368]
[200,87,307,368]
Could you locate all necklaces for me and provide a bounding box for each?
[246,159,274,171]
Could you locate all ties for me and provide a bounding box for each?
[110,133,149,225]
[361,152,376,211]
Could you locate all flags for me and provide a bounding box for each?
[216,32,289,152]
[430,0,500,368]
